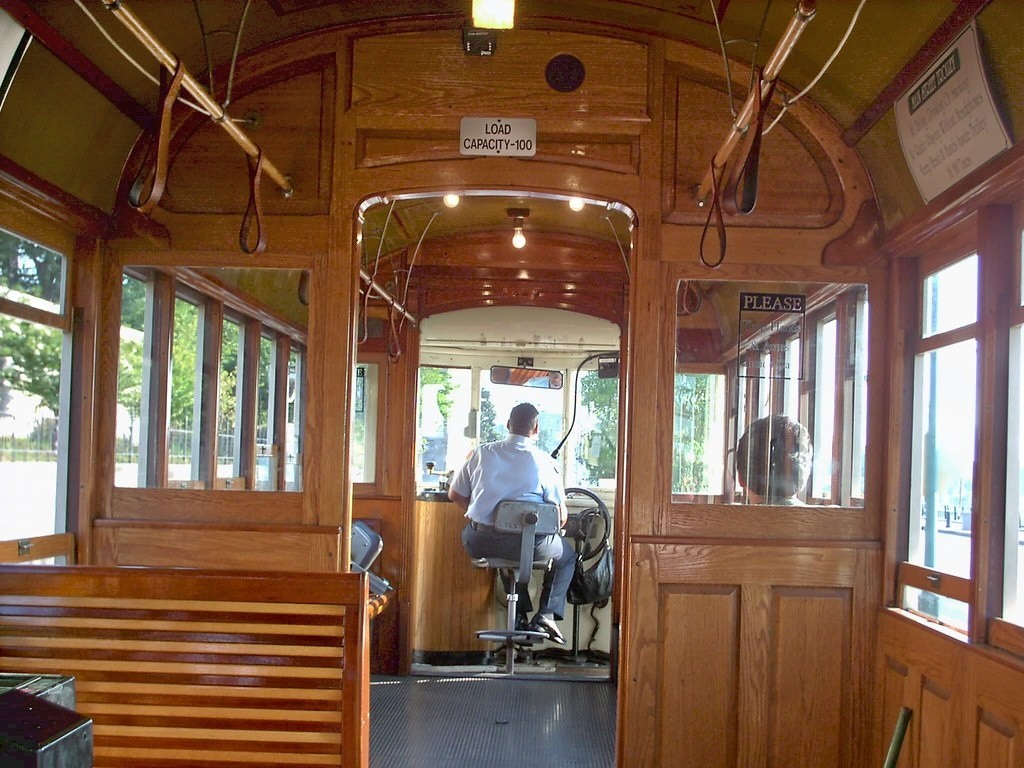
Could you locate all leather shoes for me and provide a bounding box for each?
[527,614,567,645]
[514,613,528,631]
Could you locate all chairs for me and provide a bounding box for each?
[468,499,567,675]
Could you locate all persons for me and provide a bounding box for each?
[447,401,579,646]
[735,412,815,508]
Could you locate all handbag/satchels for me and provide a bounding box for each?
[567,546,614,605]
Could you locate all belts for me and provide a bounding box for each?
[470,520,494,532]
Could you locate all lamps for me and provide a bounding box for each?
[471,0,516,30]
